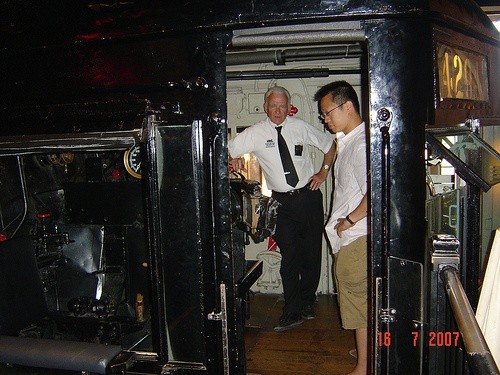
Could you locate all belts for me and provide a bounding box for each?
[273,180,312,197]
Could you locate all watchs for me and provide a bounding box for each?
[322,164,330,171]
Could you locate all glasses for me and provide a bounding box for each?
[321,101,347,119]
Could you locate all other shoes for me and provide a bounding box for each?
[273,312,304,332]
[299,304,315,319]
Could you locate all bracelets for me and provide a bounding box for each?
[346,215,356,226]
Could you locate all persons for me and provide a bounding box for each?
[228,86,337,331]
[313,81,368,375]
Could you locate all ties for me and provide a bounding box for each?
[275,126,299,188]
